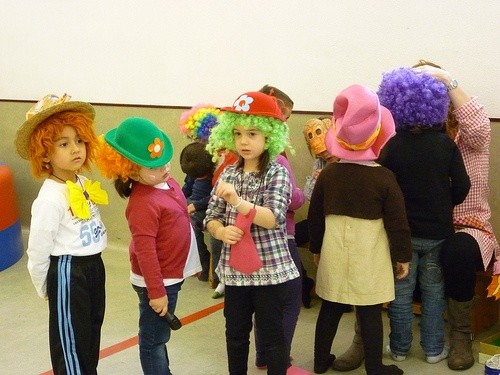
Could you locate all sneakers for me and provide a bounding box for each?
[427,347,448,363]
[386,345,406,362]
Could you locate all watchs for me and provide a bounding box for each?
[447,80,458,92]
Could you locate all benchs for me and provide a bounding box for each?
[202,229,500,338]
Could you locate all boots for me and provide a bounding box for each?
[448,295,475,369]
[332,318,365,371]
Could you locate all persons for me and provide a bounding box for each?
[376,68,471,365]
[179,106,224,299]
[97,116,201,375]
[13,94,108,375]
[202,90,301,375]
[295,117,355,313]
[331,62,500,372]
[307,84,413,375]
[214,85,309,375]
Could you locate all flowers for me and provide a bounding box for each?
[148,138,165,159]
[234,94,256,111]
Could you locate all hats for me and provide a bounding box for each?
[325,84,395,160]
[14,93,96,160]
[220,92,286,121]
[104,117,174,168]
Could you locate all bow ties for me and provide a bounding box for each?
[50,172,109,219]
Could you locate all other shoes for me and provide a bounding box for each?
[368,364,404,375]
[313,354,336,373]
[302,275,314,309]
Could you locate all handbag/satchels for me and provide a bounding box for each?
[230,204,263,275]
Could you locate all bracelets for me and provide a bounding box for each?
[232,197,242,208]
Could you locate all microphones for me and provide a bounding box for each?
[146,294,181,329]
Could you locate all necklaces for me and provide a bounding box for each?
[51,172,108,219]
